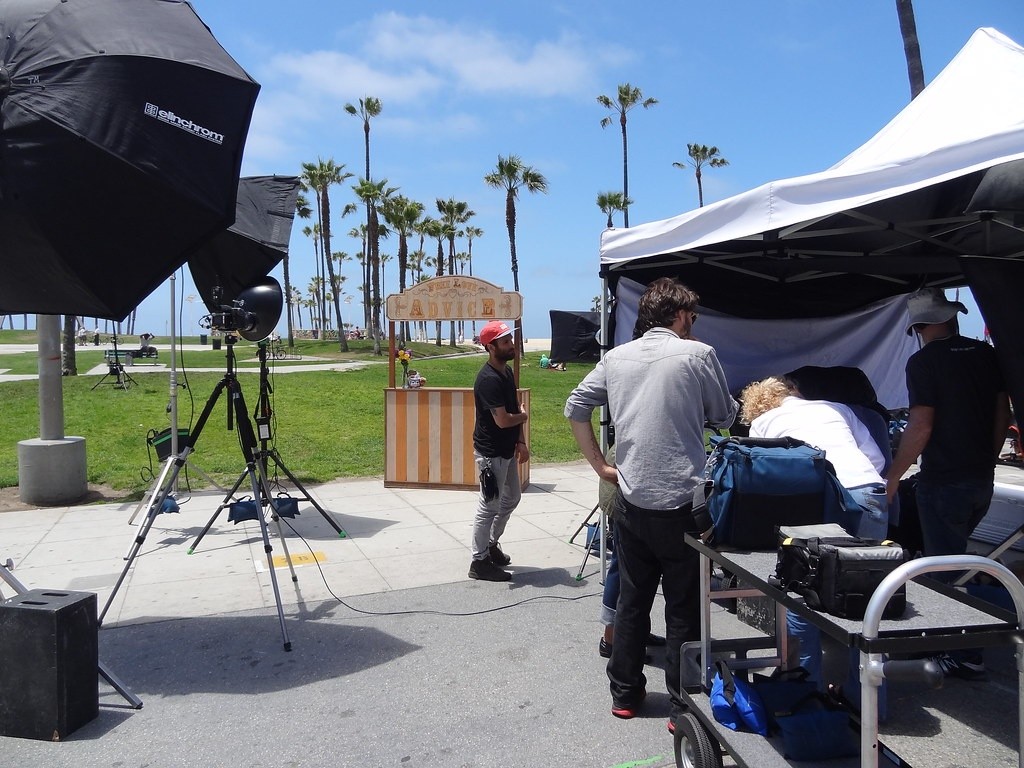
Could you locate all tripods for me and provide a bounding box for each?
[569,505,599,580]
[91,269,345,651]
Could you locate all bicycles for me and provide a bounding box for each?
[259,339,287,361]
[75,335,126,345]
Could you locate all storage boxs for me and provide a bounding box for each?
[736,575,776,638]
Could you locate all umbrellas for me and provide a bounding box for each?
[186,176,303,327]
[0,0,261,324]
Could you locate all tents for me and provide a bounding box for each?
[600,24,1023,588]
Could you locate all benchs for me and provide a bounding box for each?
[104,349,158,366]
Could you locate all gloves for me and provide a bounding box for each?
[480,467,499,503]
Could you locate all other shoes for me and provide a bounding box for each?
[599,637,653,664]
[645,633,666,646]
[611,686,646,717]
[668,721,676,735]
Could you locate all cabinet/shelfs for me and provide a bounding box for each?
[674,529,1024,768]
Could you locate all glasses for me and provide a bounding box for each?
[689,310,699,325]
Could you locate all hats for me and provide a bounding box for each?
[903,286,967,336]
[480,325,521,346]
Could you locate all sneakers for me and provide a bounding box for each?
[469,555,512,581]
[923,652,986,681]
[489,544,510,565]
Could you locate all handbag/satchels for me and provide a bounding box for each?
[710,658,772,738]
[747,666,818,731]
[768,537,910,620]
[769,691,860,761]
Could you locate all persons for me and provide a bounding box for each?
[468,322,530,581]
[140,333,156,358]
[78,326,100,346]
[540,354,551,368]
[564,276,1015,736]
[354,326,361,337]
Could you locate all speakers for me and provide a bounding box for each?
[0,588,98,741]
